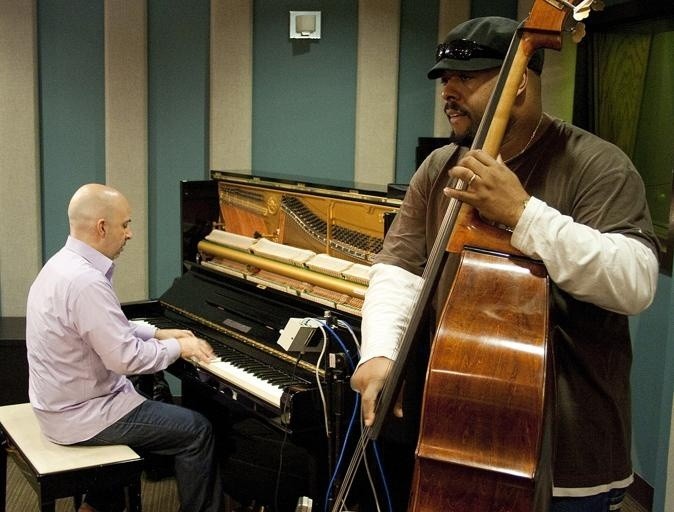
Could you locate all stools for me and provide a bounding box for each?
[1,402,144,511]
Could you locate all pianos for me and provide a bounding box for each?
[119,170,409,512]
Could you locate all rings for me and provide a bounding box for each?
[468,174,475,185]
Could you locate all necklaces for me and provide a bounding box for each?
[501,112,545,165]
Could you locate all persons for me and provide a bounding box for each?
[348,16,660,510]
[23,183,228,511]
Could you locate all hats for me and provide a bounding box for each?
[425,14,549,81]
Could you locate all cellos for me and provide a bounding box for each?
[409,0,599,511]
